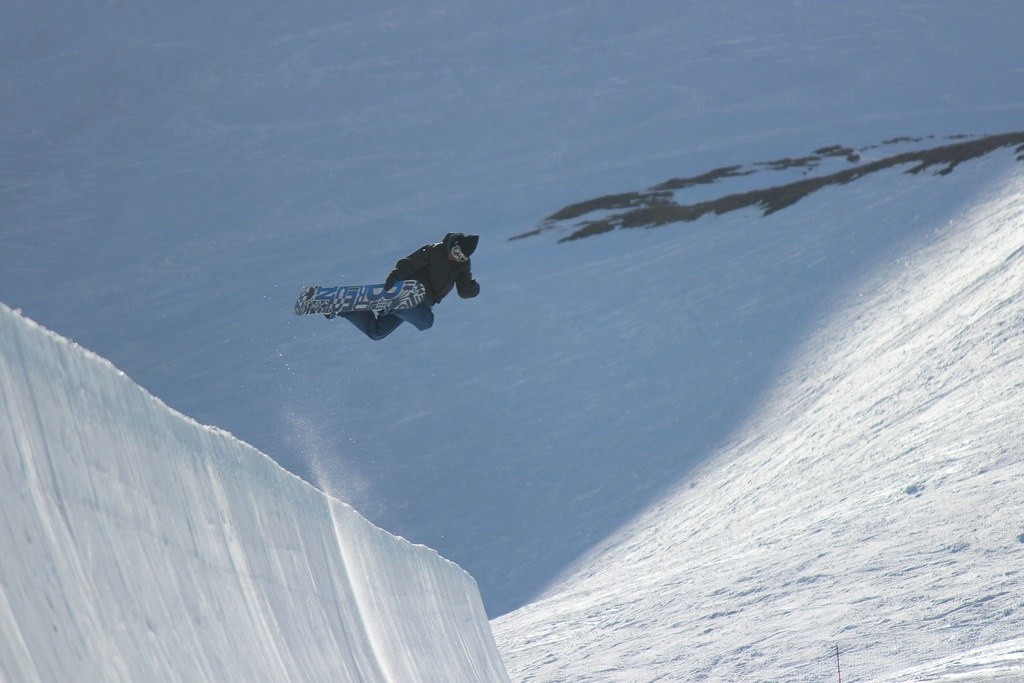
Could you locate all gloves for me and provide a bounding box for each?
[385,274,395,290]
[470,279,479,296]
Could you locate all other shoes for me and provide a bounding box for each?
[325,313,336,321]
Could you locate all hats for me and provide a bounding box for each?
[456,235,479,257]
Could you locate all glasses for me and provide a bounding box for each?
[452,247,468,263]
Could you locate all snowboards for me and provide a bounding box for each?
[294,279,426,316]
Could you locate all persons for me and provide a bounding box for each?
[324,233,480,341]
